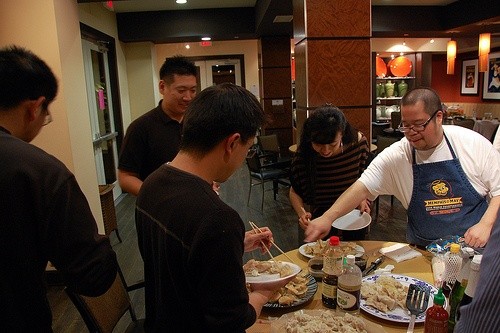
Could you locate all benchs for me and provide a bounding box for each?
[443,102,500,121]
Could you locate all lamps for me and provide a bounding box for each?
[447,38,457,74]
[479,30,490,72]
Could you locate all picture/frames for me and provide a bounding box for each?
[460,56,480,95]
[482,46,500,101]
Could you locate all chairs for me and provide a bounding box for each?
[374,113,474,218]
[63,235,145,333]
[245,134,293,214]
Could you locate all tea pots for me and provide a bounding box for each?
[376,105,400,123]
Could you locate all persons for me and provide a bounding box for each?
[0,46,119,333]
[117,54,221,197]
[303,89,500,254]
[135,81,302,333]
[287,102,370,247]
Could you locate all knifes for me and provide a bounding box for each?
[362,256,383,277]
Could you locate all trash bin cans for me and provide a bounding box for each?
[99,184,122,249]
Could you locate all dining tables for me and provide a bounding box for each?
[243,241,436,333]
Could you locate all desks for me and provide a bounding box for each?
[289,144,379,216]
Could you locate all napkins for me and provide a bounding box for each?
[380,243,422,263]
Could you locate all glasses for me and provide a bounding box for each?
[41,105,53,126]
[239,133,257,159]
[397,108,444,132]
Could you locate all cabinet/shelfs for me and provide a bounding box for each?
[371,52,422,122]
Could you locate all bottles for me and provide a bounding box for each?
[424,288,449,333]
[442,244,461,316]
[454,255,482,325]
[321,236,344,309]
[336,255,362,316]
[376,80,408,98]
[449,247,475,333]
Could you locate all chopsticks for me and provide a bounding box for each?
[301,207,323,248]
[249,221,294,269]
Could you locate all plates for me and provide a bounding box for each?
[331,209,371,230]
[376,56,412,78]
[263,270,318,307]
[359,274,447,322]
[243,260,301,285]
[271,309,387,333]
[299,241,365,258]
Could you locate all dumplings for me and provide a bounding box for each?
[286,310,370,333]
[243,259,293,278]
[268,274,308,304]
[304,241,358,257]
[360,277,420,312]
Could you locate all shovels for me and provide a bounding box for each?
[407,284,430,333]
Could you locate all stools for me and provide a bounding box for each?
[98,184,122,243]
